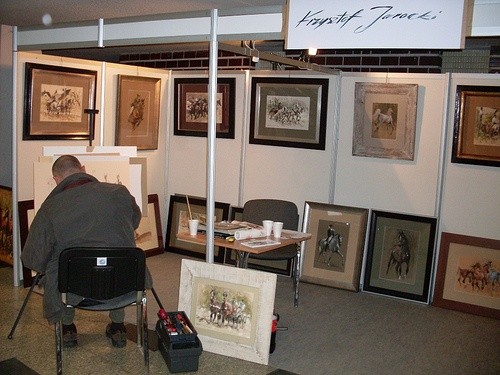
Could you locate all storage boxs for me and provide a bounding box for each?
[156,310,203,372]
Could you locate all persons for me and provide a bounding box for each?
[20,154,142,347]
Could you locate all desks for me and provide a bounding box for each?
[174,222,313,269]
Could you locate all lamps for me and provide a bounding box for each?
[300,48,320,61]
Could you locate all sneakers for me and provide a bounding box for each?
[106,323,126,348]
[62,322,77,348]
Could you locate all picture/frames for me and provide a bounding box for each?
[163,192,228,262]
[351,80,419,162]
[171,76,237,140]
[177,255,279,368]
[116,73,161,155]
[225,206,300,270]
[136,192,166,256]
[359,208,441,306]
[16,198,41,288]
[20,58,98,143]
[433,231,500,318]
[249,75,330,154]
[449,84,500,168]
[298,199,371,293]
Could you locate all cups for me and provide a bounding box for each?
[234,230,258,240]
[188,220,199,235]
[272,222,284,238]
[261,220,274,237]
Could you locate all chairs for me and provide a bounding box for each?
[42,245,151,375]
[233,196,303,291]
